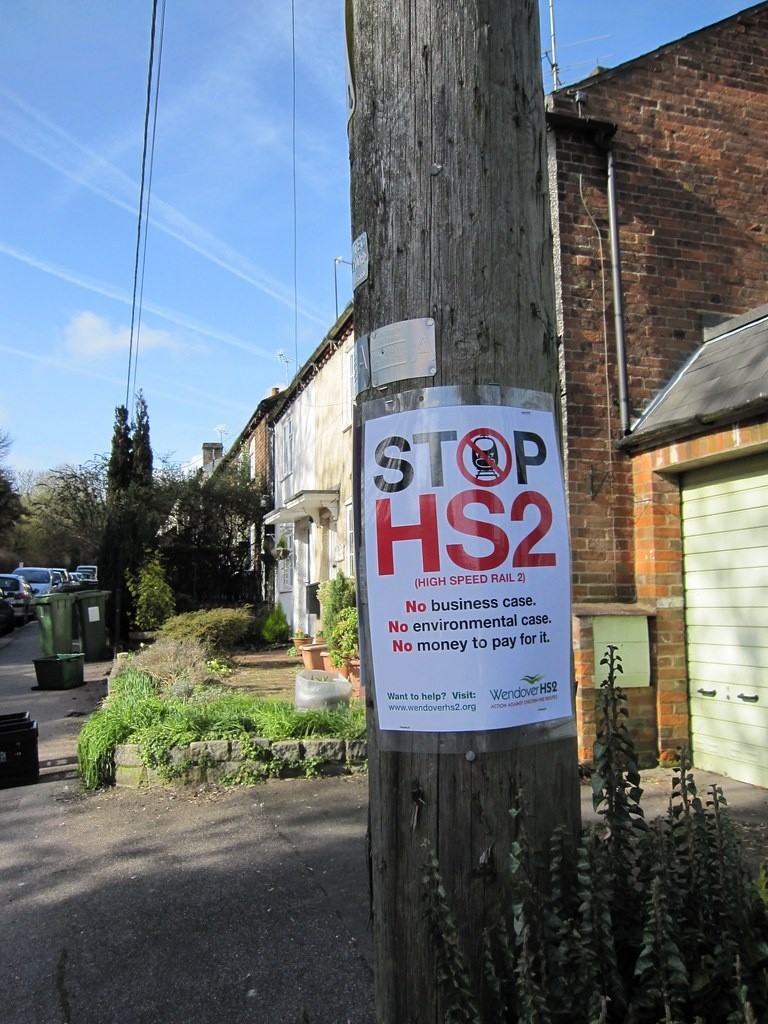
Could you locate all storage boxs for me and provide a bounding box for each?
[32,653,86,691]
[0,712,39,790]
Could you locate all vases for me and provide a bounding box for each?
[321,652,355,678]
[301,643,324,670]
[292,638,314,656]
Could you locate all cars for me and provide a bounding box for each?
[0,590,15,636]
[54,569,69,583]
[73,574,79,582]
[67,572,73,583]
[77,573,83,581]
[0,574,39,626]
[12,567,58,596]
[53,572,66,586]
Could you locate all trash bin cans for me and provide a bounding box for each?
[33,585,112,663]
[0,710,40,789]
[30,652,88,692]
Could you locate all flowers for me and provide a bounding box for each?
[292,629,310,637]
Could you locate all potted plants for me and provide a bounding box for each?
[315,630,327,643]
[270,534,290,560]
